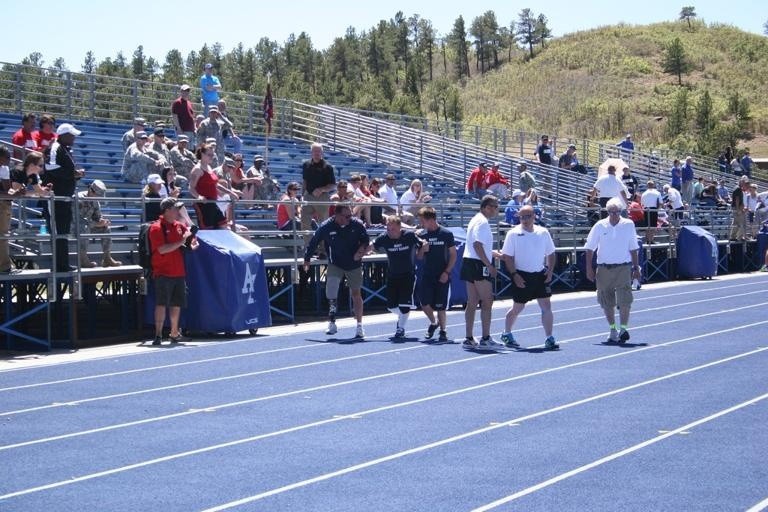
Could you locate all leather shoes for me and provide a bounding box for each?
[50,262,78,272]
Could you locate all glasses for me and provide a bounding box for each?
[518,214,534,220]
[228,166,233,168]
[157,135,164,137]
[608,211,621,216]
[134,124,144,127]
[138,138,147,140]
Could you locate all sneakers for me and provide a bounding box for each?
[152,335,163,346]
[325,321,630,351]
[256,206,262,210]
[246,205,257,210]
[758,264,768,272]
[264,205,274,210]
[167,332,193,342]
[318,253,327,260]
[0,267,22,275]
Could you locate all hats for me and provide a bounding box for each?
[542,135,548,140]
[254,154,265,163]
[223,157,236,168]
[208,105,219,114]
[204,63,214,70]
[516,161,527,167]
[133,117,149,127]
[90,178,106,197]
[493,162,499,168]
[568,144,578,151]
[56,123,82,136]
[177,135,189,143]
[479,163,491,168]
[154,119,166,128]
[159,196,185,210]
[512,189,526,197]
[180,85,194,93]
[205,137,217,147]
[153,127,167,136]
[147,173,166,185]
[135,131,148,138]
[287,181,302,190]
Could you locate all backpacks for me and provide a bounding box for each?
[138,221,152,269]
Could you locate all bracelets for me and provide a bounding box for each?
[444,269,450,276]
[510,272,518,277]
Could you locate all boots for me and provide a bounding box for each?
[101,251,122,267]
[81,255,97,268]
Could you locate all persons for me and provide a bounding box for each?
[120,61,278,228]
[693,177,732,210]
[304,204,368,338]
[672,156,693,206]
[502,204,560,349]
[149,197,200,346]
[588,165,683,227]
[583,197,642,344]
[415,206,457,342]
[277,142,432,231]
[0,113,123,274]
[459,194,506,351]
[533,135,578,201]
[466,161,542,225]
[729,179,768,241]
[616,134,634,161]
[719,147,753,178]
[359,215,424,340]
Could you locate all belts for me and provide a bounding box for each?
[596,261,632,268]
